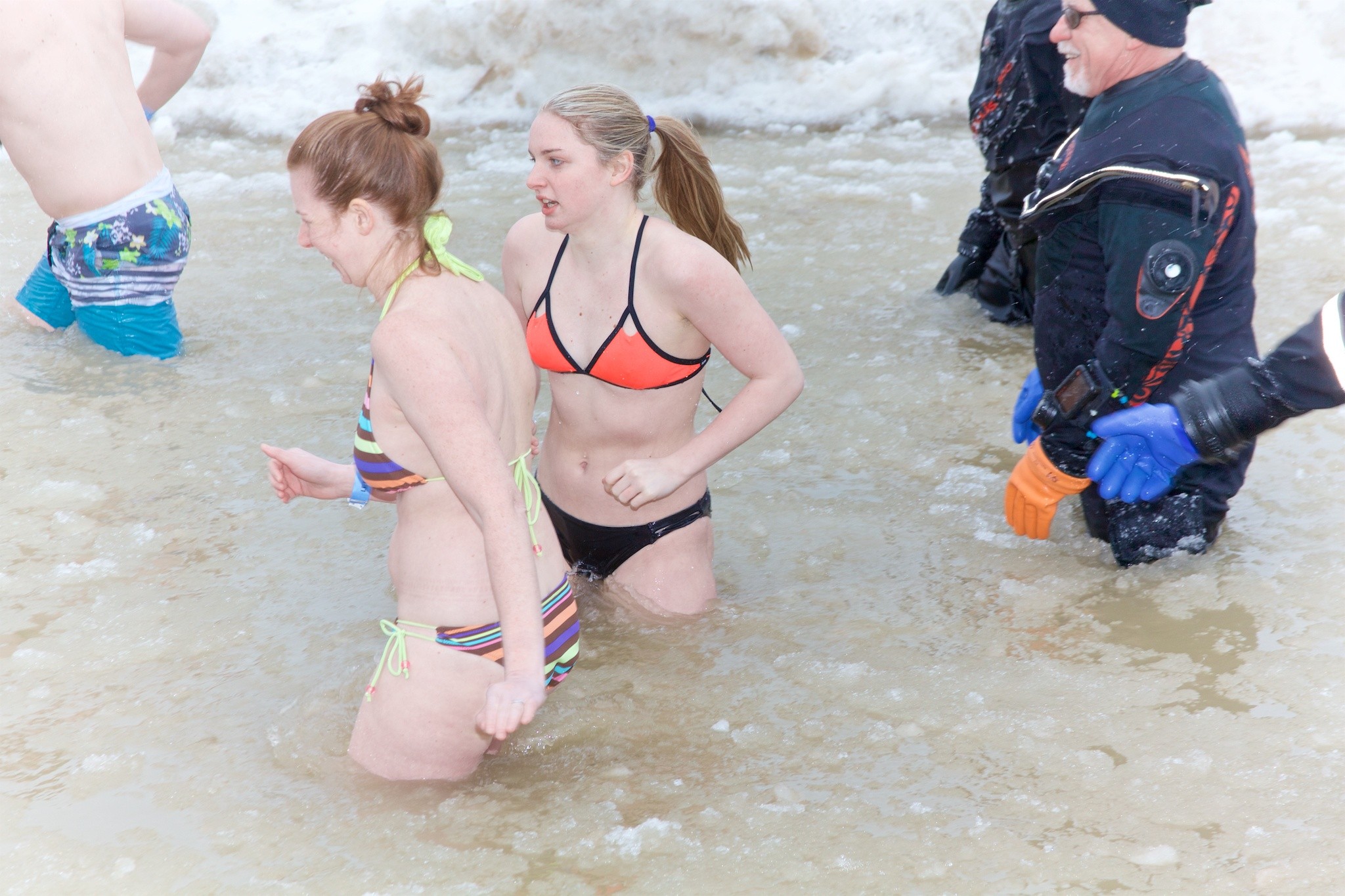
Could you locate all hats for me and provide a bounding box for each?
[1091,0,1214,49]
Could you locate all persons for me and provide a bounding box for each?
[1004,0,1260,567]
[261,67,579,780]
[501,83,805,616]
[932,0,1097,329]
[1088,289,1345,504]
[0,0,211,360]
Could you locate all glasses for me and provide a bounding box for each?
[1063,7,1101,30]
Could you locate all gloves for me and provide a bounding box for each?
[1005,437,1093,540]
[1012,367,1046,448]
[1088,402,1205,503]
[935,255,985,297]
